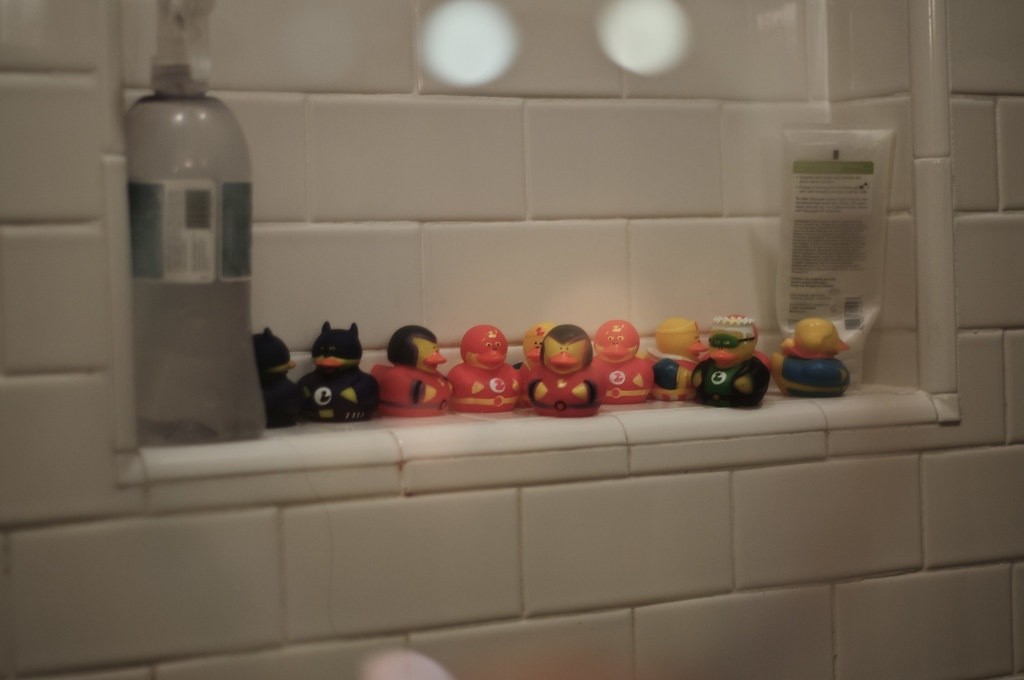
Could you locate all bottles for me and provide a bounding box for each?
[124,54,266,442]
